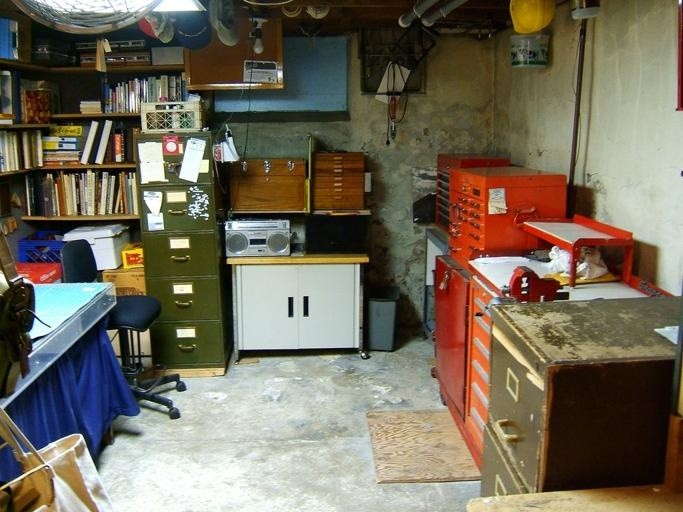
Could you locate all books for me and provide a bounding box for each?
[0,17,19,60]
[0,71,191,221]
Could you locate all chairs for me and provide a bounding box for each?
[466,288,682,512]
[61,239,186,420]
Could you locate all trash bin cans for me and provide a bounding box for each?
[367,286,400,352]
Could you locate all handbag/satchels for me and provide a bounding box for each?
[0,408,113,512]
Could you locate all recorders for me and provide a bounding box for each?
[243,59,278,83]
[223,219,291,255]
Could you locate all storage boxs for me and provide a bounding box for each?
[312,152,366,210]
[102,268,145,297]
[64,221,130,271]
[229,158,308,211]
[14,261,62,284]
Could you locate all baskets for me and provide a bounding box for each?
[18,230,66,262]
[140,100,202,134]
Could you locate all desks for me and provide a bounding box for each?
[0,283,140,484]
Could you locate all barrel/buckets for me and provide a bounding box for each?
[510,34,550,69]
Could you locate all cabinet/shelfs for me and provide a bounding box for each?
[480,296,682,498]
[184,7,284,91]
[0,0,213,221]
[227,254,370,365]
[135,126,234,367]
[447,168,567,271]
[431,255,473,416]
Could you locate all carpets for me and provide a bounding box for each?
[366,412,482,484]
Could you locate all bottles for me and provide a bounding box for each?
[155,95,180,130]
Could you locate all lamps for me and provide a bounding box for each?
[250,28,264,55]
